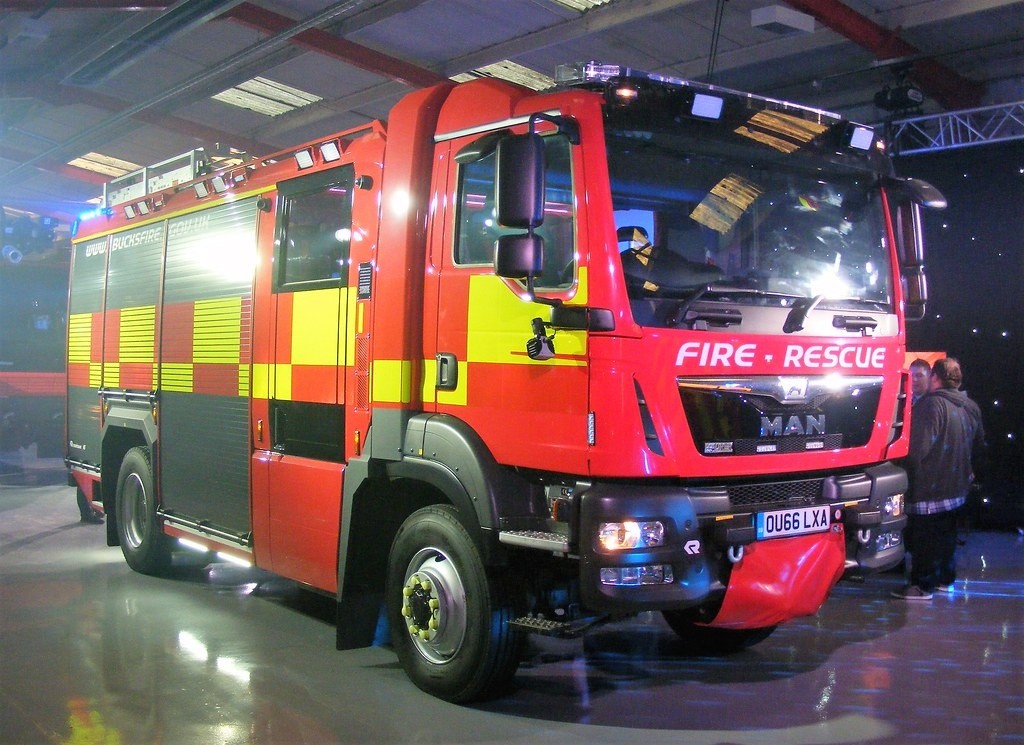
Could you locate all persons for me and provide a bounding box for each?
[78,484,106,524]
[908,359,932,410]
[886,357,980,600]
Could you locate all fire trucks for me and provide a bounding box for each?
[65,61,947,701]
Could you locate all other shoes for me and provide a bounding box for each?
[890,586,934,600]
[82,518,104,524]
